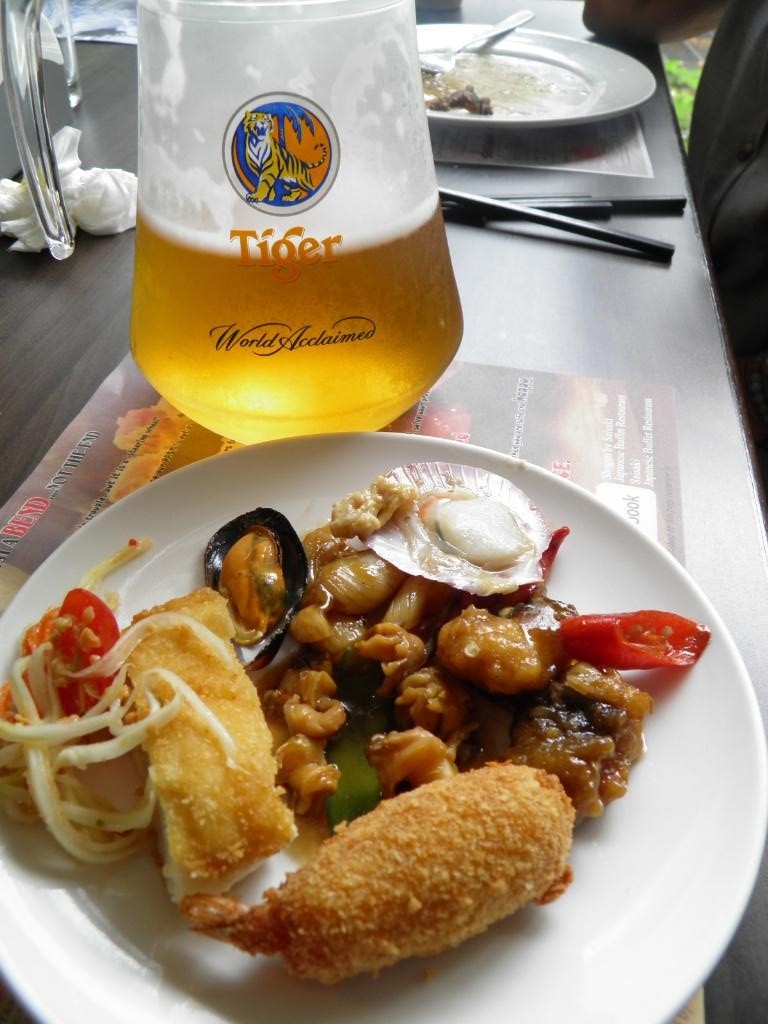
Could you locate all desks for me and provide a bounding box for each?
[0,0,768,1024]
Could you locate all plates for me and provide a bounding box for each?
[0,434,767,1023]
[410,24,657,125]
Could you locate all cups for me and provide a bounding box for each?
[0,0,463,445]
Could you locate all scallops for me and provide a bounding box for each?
[366,457,551,597]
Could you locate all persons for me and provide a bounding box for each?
[583,0,768,500]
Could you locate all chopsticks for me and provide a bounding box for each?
[434,182,688,264]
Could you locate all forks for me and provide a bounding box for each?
[419,10,540,74]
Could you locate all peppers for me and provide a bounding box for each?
[47,586,710,834]
